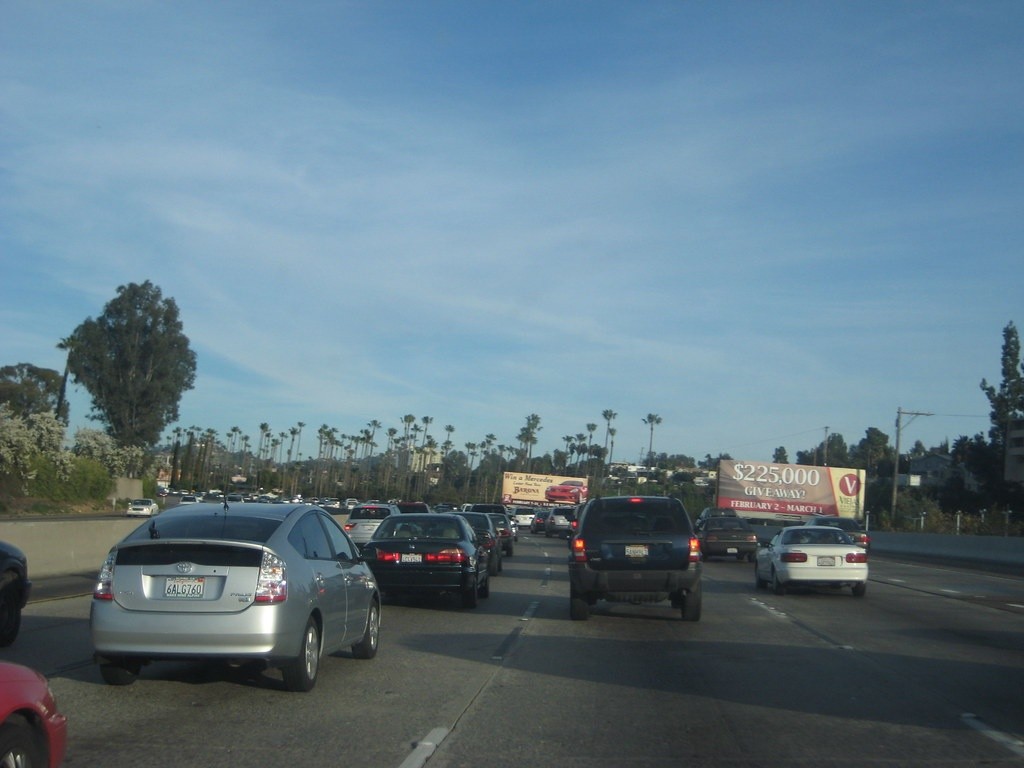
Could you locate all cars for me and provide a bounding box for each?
[90,500,383,692]
[441,511,506,576]
[694,507,758,563]
[361,514,491,609]
[125,499,158,516]
[432,504,471,512]
[435,502,520,557]
[0,542,68,768]
[742,516,804,547]
[800,517,872,550]
[755,527,869,597]
[530,512,550,535]
[545,480,588,504]
[158,489,403,510]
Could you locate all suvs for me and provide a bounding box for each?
[344,503,401,548]
[545,508,575,537]
[570,496,703,624]
[510,507,535,527]
[397,502,434,528]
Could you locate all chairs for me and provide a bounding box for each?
[441,528,458,537]
[816,533,834,543]
[651,517,674,530]
[796,533,812,542]
[396,530,412,536]
